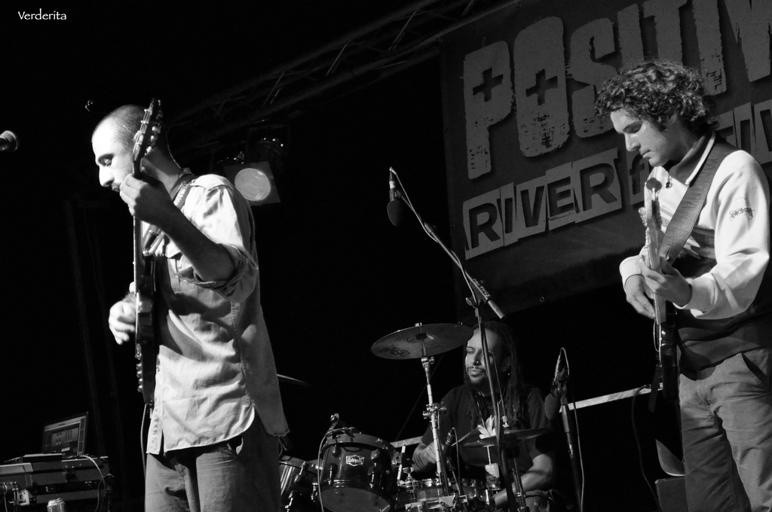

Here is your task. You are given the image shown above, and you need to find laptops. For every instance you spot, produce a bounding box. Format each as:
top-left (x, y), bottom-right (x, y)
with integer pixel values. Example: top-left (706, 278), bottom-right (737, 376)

top-left (40, 411), bottom-right (89, 459)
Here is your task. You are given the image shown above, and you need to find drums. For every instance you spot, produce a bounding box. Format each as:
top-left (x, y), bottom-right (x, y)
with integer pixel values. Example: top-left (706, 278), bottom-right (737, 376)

top-left (311, 430), bottom-right (393, 512)
top-left (397, 479), bottom-right (460, 512)
top-left (276, 452), bottom-right (316, 511)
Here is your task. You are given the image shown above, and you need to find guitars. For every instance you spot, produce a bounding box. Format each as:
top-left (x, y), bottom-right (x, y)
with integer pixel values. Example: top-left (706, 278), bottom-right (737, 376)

top-left (638, 176), bottom-right (679, 425)
top-left (130, 96), bottom-right (164, 407)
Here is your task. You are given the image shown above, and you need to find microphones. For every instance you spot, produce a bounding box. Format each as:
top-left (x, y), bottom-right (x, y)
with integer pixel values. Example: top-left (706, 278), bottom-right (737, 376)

top-left (544, 367), bottom-right (568, 421)
top-left (387, 169), bottom-right (409, 228)
top-left (443, 431), bottom-right (454, 458)
top-left (0, 129), bottom-right (22, 152)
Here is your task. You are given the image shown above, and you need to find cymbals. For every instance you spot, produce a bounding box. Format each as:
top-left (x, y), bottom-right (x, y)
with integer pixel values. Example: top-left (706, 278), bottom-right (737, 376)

top-left (461, 426), bottom-right (546, 449)
top-left (370, 322), bottom-right (475, 361)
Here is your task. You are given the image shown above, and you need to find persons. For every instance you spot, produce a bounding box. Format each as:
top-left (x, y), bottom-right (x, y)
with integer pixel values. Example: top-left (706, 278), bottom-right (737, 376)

top-left (408, 318), bottom-right (557, 512)
top-left (90, 101), bottom-right (295, 511)
top-left (590, 56), bottom-right (770, 512)
